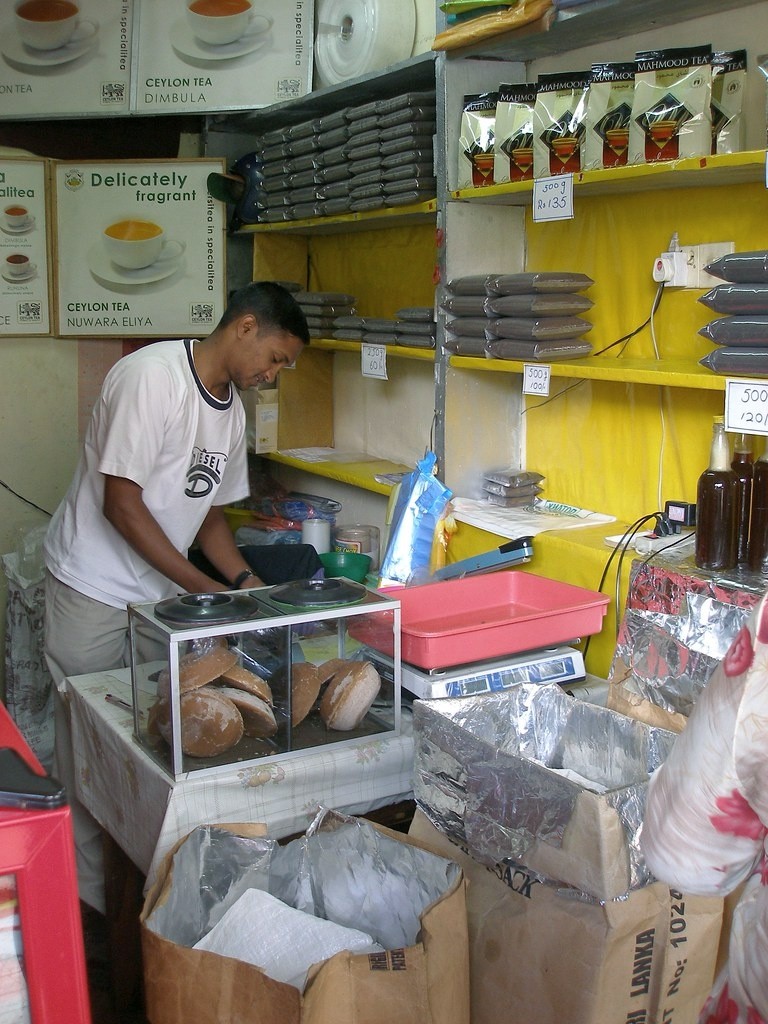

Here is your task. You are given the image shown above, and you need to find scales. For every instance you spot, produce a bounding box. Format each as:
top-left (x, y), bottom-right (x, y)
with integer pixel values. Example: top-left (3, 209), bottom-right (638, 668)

top-left (357, 640), bottom-right (588, 715)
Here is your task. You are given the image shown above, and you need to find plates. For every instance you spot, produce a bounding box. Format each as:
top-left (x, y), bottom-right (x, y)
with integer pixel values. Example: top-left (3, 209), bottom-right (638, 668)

top-left (2, 268), bottom-right (37, 281)
top-left (0, 11), bottom-right (98, 66)
top-left (168, 14), bottom-right (271, 60)
top-left (88, 240), bottom-right (182, 286)
top-left (0, 214), bottom-right (33, 233)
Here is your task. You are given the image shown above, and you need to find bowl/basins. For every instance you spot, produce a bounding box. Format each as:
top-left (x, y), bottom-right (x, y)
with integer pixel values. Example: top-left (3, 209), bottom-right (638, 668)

top-left (317, 552), bottom-right (371, 583)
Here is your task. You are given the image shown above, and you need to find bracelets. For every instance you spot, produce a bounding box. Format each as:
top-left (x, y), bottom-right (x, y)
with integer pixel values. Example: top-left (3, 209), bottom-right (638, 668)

top-left (233, 568), bottom-right (257, 587)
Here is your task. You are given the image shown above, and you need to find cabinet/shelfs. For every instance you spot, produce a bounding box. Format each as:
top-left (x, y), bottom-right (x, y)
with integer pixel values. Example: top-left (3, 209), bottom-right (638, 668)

top-left (236, 0), bottom-right (767, 601)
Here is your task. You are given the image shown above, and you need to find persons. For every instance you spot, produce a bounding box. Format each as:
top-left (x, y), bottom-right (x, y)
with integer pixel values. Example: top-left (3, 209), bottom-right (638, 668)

top-left (643, 581), bottom-right (768, 1024)
top-left (44, 281), bottom-right (311, 972)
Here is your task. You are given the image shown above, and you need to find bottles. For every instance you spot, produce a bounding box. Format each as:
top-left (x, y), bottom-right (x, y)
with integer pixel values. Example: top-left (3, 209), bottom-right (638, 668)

top-left (695, 415), bottom-right (739, 570)
top-left (335, 523), bottom-right (379, 570)
top-left (729, 430), bottom-right (754, 563)
top-left (746, 450), bottom-right (768, 573)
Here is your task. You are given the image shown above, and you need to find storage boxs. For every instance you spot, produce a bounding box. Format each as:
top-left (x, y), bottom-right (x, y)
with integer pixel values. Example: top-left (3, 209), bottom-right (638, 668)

top-left (348, 570), bottom-right (610, 667)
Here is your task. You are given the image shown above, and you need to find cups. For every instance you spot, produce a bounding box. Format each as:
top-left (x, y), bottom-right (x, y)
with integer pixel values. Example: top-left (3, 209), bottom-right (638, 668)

top-left (14, 0), bottom-right (100, 50)
top-left (103, 219), bottom-right (187, 268)
top-left (185, 0), bottom-right (273, 44)
top-left (7, 254), bottom-right (38, 274)
top-left (2, 204), bottom-right (35, 227)
